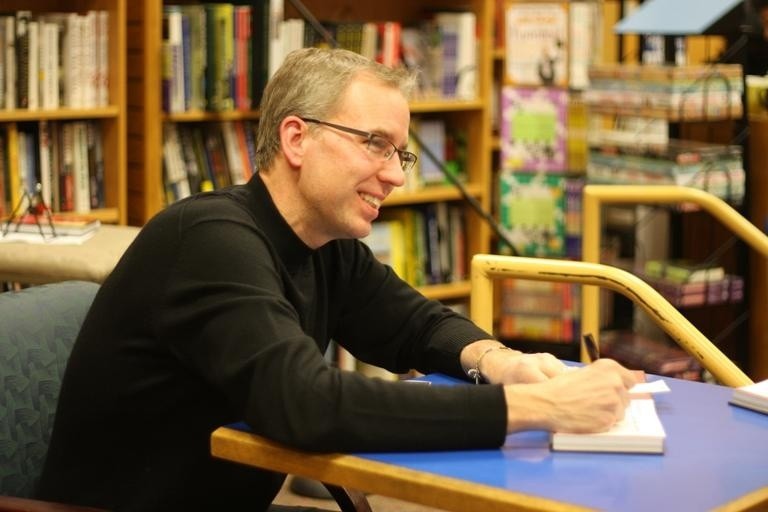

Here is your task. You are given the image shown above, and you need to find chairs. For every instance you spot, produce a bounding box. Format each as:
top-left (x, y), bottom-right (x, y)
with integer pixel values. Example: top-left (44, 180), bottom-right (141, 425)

top-left (0, 280), bottom-right (110, 511)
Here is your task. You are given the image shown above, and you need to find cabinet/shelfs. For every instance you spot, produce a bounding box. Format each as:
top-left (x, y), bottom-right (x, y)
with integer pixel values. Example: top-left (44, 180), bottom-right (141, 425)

top-left (127, 0), bottom-right (492, 321)
top-left (579, 72), bottom-right (749, 311)
top-left (0, 0), bottom-right (127, 228)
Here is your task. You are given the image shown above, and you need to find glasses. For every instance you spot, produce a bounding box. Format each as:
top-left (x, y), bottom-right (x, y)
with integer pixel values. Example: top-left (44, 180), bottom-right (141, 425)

top-left (300, 117), bottom-right (417, 173)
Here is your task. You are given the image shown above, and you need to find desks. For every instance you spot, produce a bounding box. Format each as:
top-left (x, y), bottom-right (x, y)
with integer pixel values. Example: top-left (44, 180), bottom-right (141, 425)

top-left (211, 360), bottom-right (767, 512)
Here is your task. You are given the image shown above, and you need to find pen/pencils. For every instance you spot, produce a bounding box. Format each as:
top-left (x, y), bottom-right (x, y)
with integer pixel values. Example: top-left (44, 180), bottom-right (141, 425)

top-left (584, 335), bottom-right (599, 361)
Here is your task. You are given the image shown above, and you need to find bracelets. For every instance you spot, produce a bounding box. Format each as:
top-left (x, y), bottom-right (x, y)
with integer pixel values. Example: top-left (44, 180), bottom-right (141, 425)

top-left (466, 344), bottom-right (517, 384)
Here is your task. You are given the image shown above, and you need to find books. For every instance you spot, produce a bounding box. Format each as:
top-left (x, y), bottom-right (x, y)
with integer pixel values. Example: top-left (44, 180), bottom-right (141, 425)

top-left (163, 121), bottom-right (258, 206)
top-left (0, 121), bottom-right (105, 224)
top-left (1, 11), bottom-right (109, 109)
top-left (356, 203), bottom-right (471, 285)
top-left (642, 0), bottom-right (687, 66)
top-left (162, 0), bottom-right (480, 114)
top-left (547, 367), bottom-right (666, 456)
top-left (499, 1), bottom-right (605, 338)
top-left (400, 117), bottom-right (445, 189)
top-left (582, 62), bottom-right (745, 385)
top-left (726, 377), bottom-right (768, 416)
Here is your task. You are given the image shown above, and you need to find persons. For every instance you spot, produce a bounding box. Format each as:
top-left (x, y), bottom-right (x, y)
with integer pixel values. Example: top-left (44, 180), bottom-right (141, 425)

top-left (37, 46), bottom-right (640, 512)
